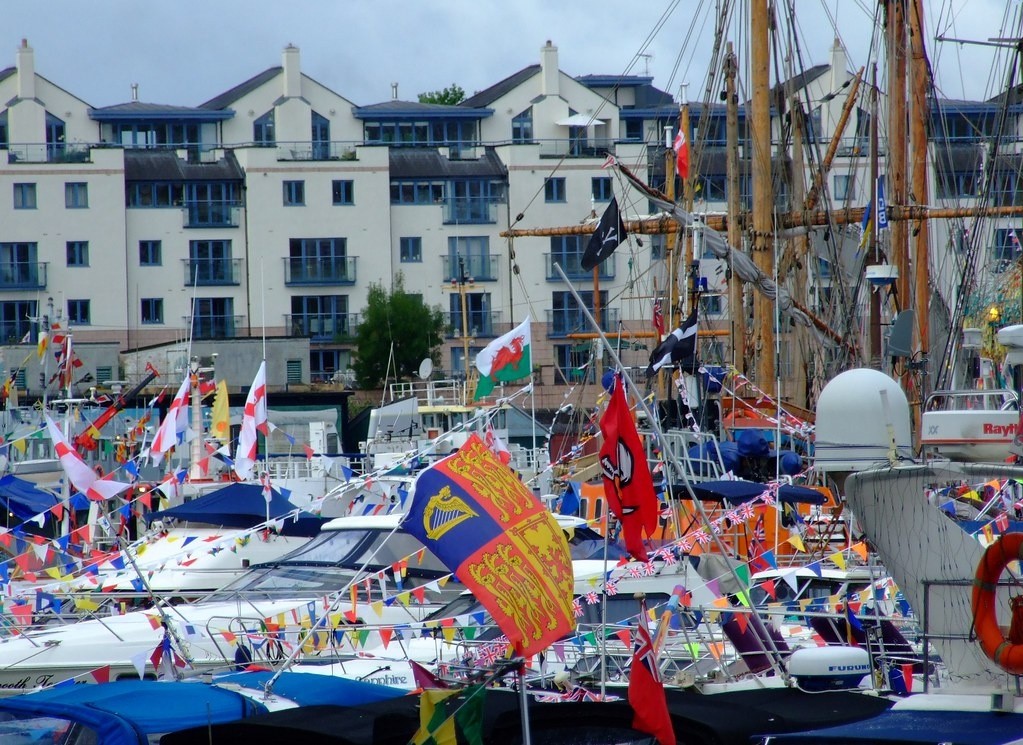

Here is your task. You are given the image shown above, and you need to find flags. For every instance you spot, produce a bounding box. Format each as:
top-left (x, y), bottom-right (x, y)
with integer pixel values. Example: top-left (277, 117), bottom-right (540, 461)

top-left (691, 258), bottom-right (728, 291)
top-left (407, 659), bottom-right (485, 745)
top-left (854, 174), bottom-right (888, 260)
top-left (672, 122), bottom-right (689, 179)
top-left (628, 600), bottom-right (676, 745)
top-left (645, 308), bottom-right (697, 377)
top-left (599, 375), bottom-right (658, 562)
top-left (43, 410), bottom-right (133, 501)
top-left (580, 197), bottom-right (628, 272)
top-left (398, 431), bottom-right (576, 658)
top-left (473, 315), bottom-right (530, 403)
top-left (149, 376), bottom-right (190, 467)
top-left (234, 360), bottom-right (267, 482)
top-left (650, 288), bottom-right (665, 342)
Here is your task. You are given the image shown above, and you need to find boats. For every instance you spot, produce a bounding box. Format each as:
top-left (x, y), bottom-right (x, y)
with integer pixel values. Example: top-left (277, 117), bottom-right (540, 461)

top-left (2, 0), bottom-right (1023, 745)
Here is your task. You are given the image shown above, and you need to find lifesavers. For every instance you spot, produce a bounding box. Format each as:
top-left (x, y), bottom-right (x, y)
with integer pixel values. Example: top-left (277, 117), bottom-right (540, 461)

top-left (970, 531), bottom-right (1022, 677)
